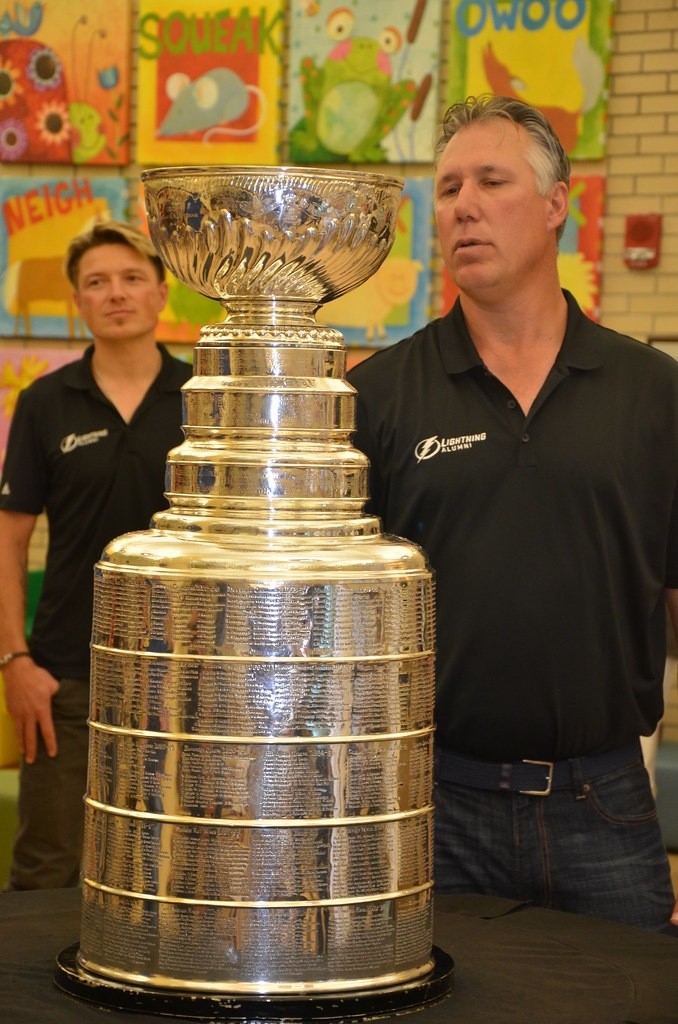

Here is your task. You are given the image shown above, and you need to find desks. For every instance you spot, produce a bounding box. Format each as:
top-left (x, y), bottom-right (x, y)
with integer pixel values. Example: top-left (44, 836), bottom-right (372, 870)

top-left (0, 887), bottom-right (678, 1024)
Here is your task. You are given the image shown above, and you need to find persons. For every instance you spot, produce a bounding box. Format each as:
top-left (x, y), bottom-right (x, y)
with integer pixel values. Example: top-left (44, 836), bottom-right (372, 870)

top-left (346, 95), bottom-right (678, 928)
top-left (0, 222), bottom-right (192, 892)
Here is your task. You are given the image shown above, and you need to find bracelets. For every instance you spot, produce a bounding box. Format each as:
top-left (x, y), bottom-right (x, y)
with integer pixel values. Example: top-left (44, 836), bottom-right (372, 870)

top-left (0, 652), bottom-right (30, 670)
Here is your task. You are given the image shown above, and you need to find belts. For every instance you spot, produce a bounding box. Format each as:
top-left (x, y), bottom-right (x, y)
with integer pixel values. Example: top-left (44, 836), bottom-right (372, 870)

top-left (435, 739), bottom-right (643, 795)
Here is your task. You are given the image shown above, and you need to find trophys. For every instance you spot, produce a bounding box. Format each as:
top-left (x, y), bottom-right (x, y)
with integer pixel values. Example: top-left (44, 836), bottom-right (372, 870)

top-left (59, 166), bottom-right (455, 1024)
